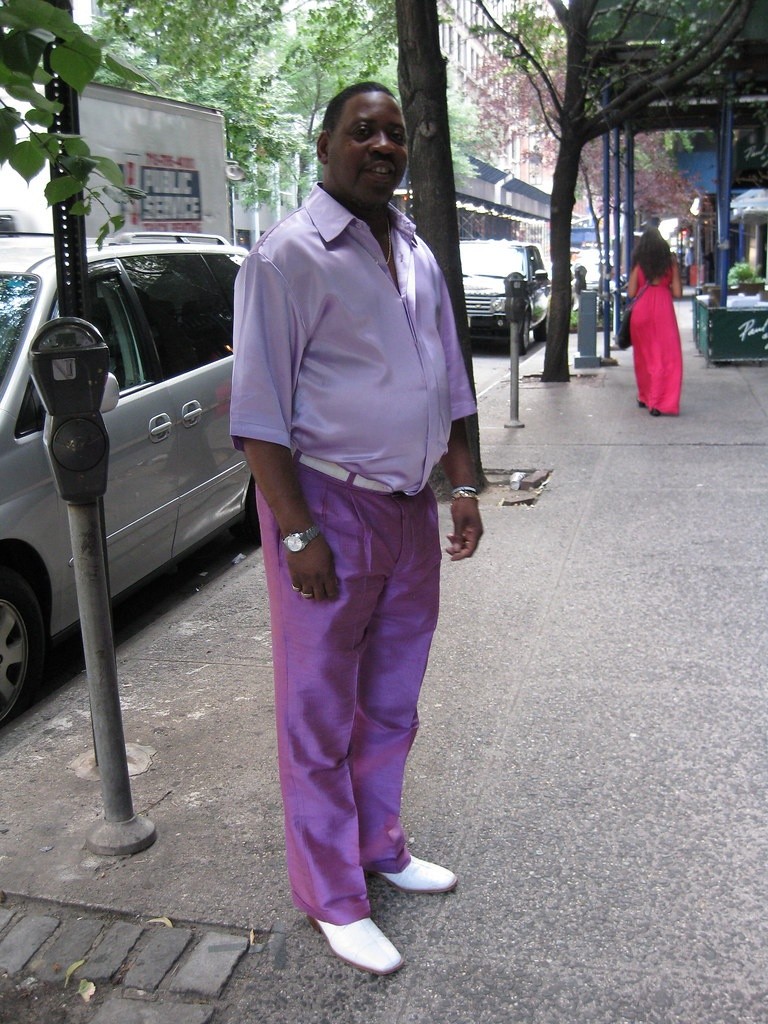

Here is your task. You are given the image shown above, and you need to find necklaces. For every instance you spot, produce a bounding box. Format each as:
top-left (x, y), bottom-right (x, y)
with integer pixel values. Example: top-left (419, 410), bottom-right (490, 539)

top-left (386, 217), bottom-right (391, 263)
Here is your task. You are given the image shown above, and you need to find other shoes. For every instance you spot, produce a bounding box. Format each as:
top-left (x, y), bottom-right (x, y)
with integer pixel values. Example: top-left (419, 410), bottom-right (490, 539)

top-left (379, 854), bottom-right (456, 894)
top-left (308, 914), bottom-right (405, 974)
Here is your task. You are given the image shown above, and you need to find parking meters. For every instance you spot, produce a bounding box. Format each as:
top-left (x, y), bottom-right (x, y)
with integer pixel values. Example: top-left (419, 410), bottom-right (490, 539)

top-left (25, 311), bottom-right (156, 854)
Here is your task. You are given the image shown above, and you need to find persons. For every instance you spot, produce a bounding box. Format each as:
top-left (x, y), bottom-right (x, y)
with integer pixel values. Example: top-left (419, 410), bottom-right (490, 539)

top-left (685, 247), bottom-right (695, 285)
top-left (230, 82), bottom-right (483, 975)
top-left (627, 228), bottom-right (682, 416)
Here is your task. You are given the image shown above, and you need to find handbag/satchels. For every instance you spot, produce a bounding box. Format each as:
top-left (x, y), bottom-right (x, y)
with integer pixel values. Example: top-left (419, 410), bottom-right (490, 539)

top-left (615, 308), bottom-right (633, 347)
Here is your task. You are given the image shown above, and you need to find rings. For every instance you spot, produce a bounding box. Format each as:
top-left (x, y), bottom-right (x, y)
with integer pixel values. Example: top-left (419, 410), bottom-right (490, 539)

top-left (291, 583), bottom-right (302, 591)
top-left (465, 541), bottom-right (469, 543)
top-left (301, 592), bottom-right (313, 598)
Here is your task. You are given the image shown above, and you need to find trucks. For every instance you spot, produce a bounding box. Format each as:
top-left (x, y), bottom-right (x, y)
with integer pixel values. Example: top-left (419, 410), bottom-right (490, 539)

top-left (0, 76), bottom-right (237, 253)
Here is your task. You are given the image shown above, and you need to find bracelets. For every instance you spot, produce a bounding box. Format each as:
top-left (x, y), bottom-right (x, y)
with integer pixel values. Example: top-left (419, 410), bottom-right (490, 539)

top-left (451, 486), bottom-right (478, 499)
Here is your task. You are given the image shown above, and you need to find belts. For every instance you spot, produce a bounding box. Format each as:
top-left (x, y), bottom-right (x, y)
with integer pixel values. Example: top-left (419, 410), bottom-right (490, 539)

top-left (292, 452), bottom-right (401, 497)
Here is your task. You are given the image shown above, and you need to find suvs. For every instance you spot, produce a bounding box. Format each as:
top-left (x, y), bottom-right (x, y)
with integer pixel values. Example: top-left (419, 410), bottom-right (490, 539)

top-left (1, 228), bottom-right (257, 738)
top-left (456, 238), bottom-right (555, 357)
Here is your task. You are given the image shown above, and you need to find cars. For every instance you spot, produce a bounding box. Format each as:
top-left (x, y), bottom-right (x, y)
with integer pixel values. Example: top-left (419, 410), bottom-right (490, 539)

top-left (568, 246), bottom-right (614, 292)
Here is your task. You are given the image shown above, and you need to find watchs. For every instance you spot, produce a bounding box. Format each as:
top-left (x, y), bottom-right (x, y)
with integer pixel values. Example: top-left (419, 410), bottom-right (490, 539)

top-left (281, 525), bottom-right (319, 551)
